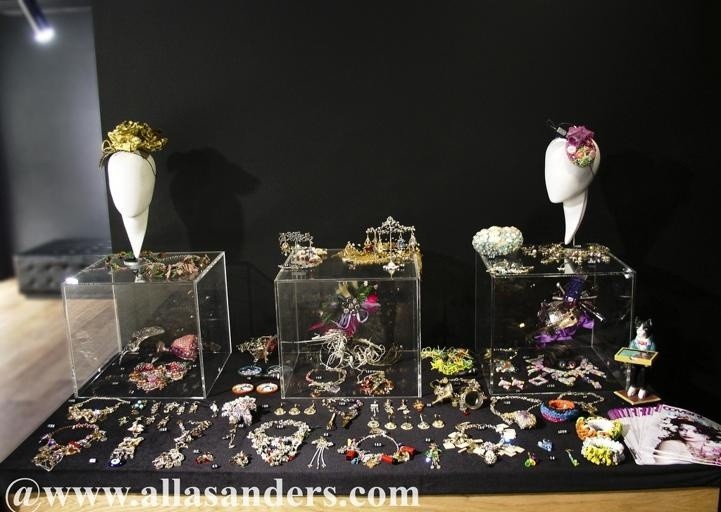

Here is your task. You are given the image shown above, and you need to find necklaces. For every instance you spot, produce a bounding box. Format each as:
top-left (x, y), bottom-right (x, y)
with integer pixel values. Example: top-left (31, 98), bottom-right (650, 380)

top-left (68, 397), bottom-right (131, 424)
top-left (555, 391), bottom-right (606, 418)
top-left (441, 421), bottom-right (527, 466)
top-left (489, 395), bottom-right (542, 430)
top-left (32, 422), bottom-right (108, 473)
top-left (337, 434), bottom-right (417, 471)
top-left (245, 419), bottom-right (311, 467)
top-left (306, 367), bottom-right (347, 398)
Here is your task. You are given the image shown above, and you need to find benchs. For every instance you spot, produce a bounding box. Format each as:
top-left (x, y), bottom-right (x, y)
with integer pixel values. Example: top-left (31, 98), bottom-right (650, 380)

top-left (12, 236), bottom-right (117, 301)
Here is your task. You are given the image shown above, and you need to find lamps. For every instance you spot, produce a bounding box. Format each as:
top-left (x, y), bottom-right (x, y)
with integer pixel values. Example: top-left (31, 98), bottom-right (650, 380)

top-left (18, 0), bottom-right (55, 44)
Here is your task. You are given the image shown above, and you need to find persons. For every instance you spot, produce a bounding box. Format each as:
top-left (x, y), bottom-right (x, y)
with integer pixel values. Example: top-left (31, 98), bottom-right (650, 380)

top-left (656, 417), bottom-right (719, 461)
top-left (623, 316), bottom-right (655, 400)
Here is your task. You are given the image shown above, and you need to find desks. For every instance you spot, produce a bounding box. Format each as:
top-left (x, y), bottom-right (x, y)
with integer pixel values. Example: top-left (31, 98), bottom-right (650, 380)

top-left (0, 349), bottom-right (721, 512)
